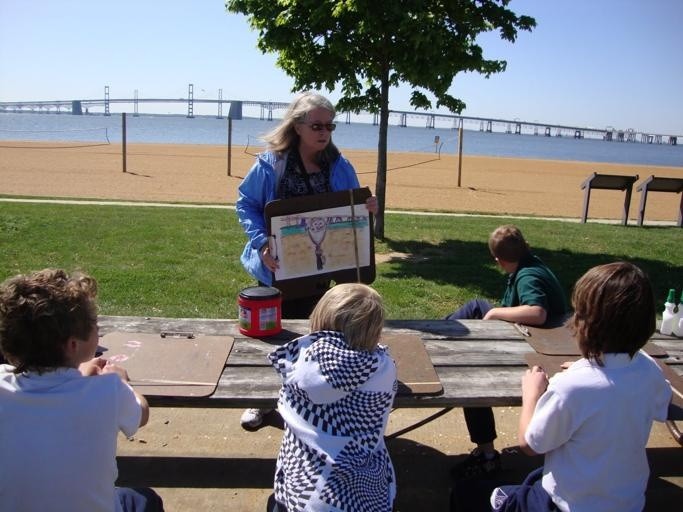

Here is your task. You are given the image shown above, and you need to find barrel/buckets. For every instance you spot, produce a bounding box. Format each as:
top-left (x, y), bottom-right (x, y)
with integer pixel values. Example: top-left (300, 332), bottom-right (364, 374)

top-left (237, 286), bottom-right (282, 337)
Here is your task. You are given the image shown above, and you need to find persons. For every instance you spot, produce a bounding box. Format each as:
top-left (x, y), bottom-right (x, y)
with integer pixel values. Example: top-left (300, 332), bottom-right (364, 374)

top-left (450, 262), bottom-right (676, 512)
top-left (443, 225), bottom-right (567, 472)
top-left (267, 282), bottom-right (399, 511)
top-left (0, 267), bottom-right (166, 512)
top-left (234, 91), bottom-right (379, 430)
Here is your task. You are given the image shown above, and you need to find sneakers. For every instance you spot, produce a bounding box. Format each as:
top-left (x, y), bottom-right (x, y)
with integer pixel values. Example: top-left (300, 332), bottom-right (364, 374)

top-left (451, 448), bottom-right (503, 484)
top-left (239, 406), bottom-right (274, 432)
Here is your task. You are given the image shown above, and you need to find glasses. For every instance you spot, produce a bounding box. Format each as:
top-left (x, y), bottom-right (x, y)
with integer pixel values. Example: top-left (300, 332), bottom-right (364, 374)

top-left (309, 123), bottom-right (336, 132)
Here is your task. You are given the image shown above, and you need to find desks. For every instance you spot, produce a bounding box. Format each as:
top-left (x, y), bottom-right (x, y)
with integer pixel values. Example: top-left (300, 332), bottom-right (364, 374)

top-left (85, 308), bottom-right (683, 468)
